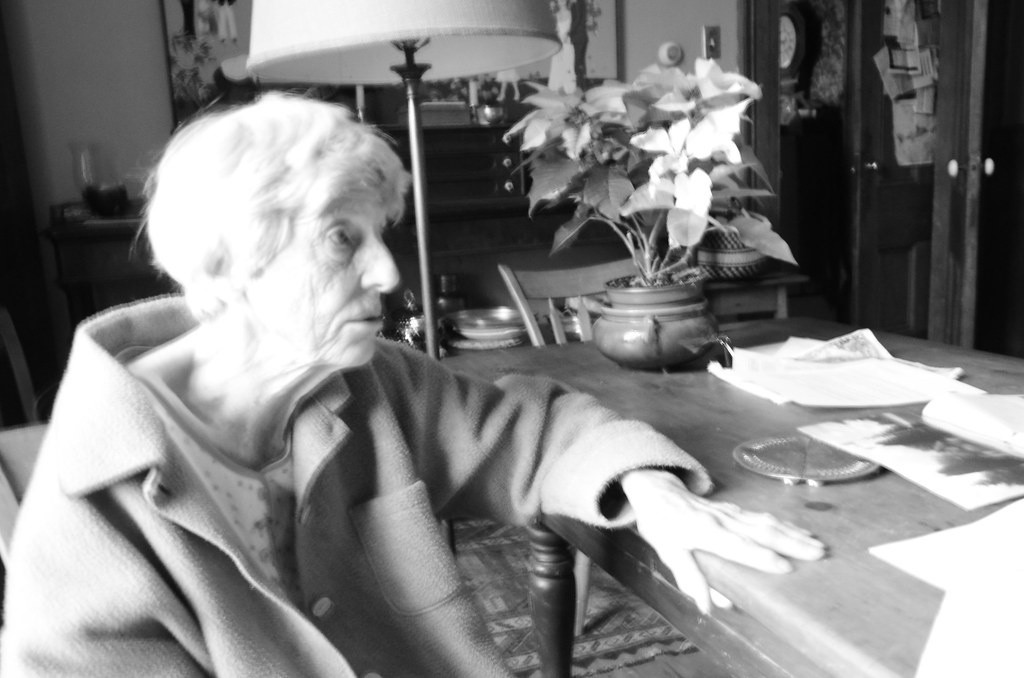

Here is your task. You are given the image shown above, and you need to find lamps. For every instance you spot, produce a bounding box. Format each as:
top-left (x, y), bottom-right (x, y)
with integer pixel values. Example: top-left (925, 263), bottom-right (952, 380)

top-left (246, 1), bottom-right (564, 355)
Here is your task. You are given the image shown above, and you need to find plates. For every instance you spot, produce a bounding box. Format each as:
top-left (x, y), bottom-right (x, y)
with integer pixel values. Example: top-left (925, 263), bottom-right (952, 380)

top-left (734, 437), bottom-right (880, 480)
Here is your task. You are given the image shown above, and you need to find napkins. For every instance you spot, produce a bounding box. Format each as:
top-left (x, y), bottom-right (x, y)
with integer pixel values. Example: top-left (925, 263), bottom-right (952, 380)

top-left (706, 328), bottom-right (1024, 589)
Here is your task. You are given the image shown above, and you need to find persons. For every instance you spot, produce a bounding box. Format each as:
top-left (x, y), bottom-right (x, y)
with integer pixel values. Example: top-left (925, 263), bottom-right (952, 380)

top-left (0, 89), bottom-right (830, 678)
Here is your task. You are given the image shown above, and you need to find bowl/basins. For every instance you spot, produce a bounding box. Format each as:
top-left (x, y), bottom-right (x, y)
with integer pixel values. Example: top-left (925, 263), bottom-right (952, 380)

top-left (444, 307), bottom-right (537, 340)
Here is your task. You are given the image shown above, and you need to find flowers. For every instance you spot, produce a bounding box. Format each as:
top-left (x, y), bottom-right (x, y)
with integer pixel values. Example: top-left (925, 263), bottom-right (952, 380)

top-left (512, 57), bottom-right (799, 286)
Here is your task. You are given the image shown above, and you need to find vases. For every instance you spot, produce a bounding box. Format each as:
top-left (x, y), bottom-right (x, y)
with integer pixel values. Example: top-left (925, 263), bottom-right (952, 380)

top-left (593, 269), bottom-right (721, 372)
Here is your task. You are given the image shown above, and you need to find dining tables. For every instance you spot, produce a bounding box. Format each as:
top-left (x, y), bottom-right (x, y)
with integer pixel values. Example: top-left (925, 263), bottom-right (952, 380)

top-left (427, 315), bottom-right (1024, 677)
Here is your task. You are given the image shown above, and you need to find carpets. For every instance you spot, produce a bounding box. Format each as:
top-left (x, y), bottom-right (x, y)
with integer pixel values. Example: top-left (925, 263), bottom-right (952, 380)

top-left (437, 518), bottom-right (698, 677)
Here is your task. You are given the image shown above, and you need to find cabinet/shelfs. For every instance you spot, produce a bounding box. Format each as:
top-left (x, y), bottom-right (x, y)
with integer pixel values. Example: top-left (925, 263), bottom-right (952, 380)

top-left (52, 199), bottom-right (181, 328)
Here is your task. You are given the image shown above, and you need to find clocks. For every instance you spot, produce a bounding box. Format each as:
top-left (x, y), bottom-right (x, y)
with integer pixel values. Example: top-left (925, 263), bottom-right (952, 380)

top-left (778, 0), bottom-right (816, 127)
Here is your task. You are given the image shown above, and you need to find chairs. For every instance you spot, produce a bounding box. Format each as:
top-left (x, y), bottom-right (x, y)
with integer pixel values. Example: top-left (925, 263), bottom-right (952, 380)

top-left (498, 254), bottom-right (642, 347)
